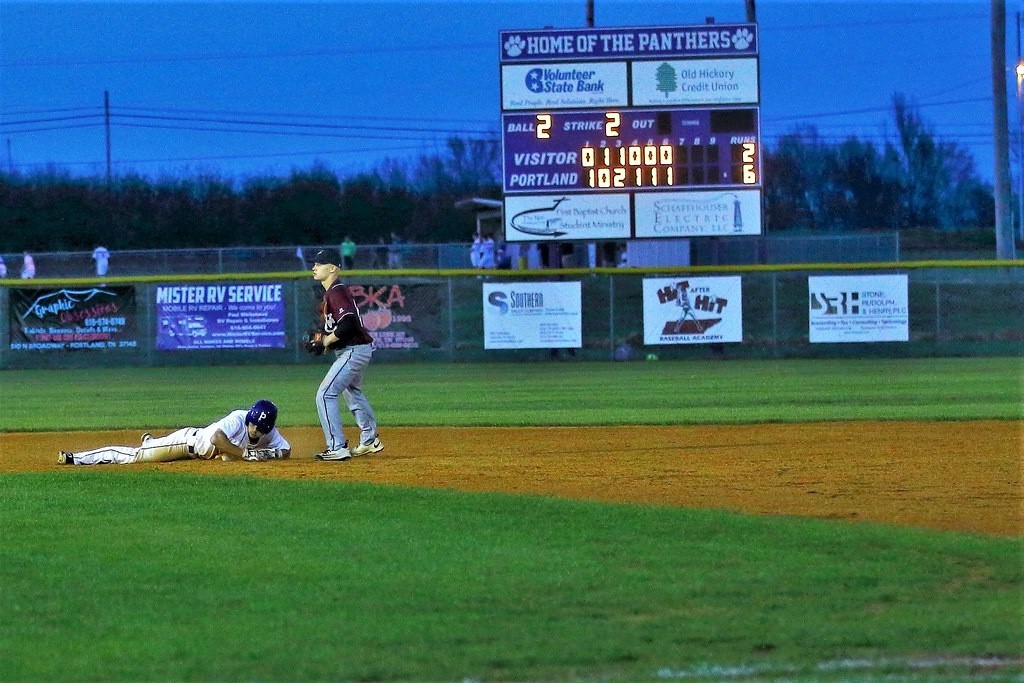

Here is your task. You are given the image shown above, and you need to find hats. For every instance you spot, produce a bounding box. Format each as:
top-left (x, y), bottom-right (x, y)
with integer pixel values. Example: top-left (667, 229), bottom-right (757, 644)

top-left (306, 248), bottom-right (342, 268)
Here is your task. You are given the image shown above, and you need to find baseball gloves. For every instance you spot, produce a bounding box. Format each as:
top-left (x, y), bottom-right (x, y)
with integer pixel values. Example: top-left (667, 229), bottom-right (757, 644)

top-left (303, 327), bottom-right (326, 356)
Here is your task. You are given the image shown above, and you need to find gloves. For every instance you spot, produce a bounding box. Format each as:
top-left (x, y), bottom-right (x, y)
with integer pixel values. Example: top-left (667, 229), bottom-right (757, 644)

top-left (242, 447), bottom-right (268, 462)
top-left (259, 447), bottom-right (283, 459)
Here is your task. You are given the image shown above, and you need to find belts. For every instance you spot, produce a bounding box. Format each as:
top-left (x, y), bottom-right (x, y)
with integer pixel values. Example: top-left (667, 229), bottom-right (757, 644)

top-left (189, 431), bottom-right (196, 454)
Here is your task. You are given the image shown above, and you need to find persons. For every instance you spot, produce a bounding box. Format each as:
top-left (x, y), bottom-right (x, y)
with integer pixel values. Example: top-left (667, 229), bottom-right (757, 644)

top-left (336, 231), bottom-right (411, 270)
top-left (304, 248), bottom-right (385, 462)
top-left (469, 232), bottom-right (632, 270)
top-left (0, 258), bottom-right (7, 278)
top-left (18, 250), bottom-right (37, 279)
top-left (92, 241), bottom-right (110, 275)
top-left (56, 399), bottom-right (292, 466)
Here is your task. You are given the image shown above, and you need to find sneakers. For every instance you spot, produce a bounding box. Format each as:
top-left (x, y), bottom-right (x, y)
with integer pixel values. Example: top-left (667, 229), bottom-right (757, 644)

top-left (141, 432), bottom-right (153, 444)
top-left (58, 450), bottom-right (74, 465)
top-left (350, 434), bottom-right (384, 457)
top-left (313, 440), bottom-right (351, 461)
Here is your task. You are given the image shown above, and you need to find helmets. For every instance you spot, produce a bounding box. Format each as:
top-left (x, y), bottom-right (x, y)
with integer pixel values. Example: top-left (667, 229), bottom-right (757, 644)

top-left (245, 400), bottom-right (277, 434)
